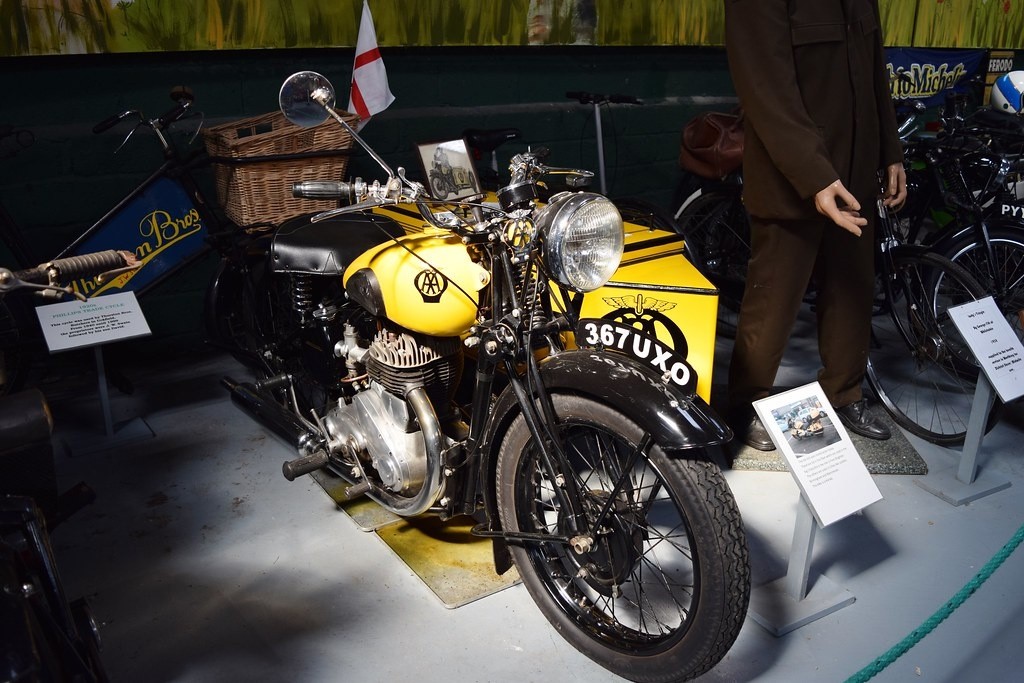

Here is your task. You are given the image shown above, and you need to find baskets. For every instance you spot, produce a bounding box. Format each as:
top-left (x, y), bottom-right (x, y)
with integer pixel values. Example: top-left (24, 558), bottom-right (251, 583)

top-left (202, 105), bottom-right (361, 233)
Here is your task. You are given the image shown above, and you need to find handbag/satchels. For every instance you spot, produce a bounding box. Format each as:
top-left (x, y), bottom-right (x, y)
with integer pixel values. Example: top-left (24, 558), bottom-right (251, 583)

top-left (678, 112), bottom-right (743, 177)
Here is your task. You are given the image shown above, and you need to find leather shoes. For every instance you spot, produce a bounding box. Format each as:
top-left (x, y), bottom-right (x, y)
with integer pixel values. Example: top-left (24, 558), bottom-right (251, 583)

top-left (727, 400), bottom-right (775, 451)
top-left (834, 399), bottom-right (891, 440)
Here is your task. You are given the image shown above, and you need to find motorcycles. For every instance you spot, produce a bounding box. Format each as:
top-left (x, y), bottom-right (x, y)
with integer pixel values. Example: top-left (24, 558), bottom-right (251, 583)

top-left (0, 248), bottom-right (144, 683)
top-left (218, 72), bottom-right (752, 683)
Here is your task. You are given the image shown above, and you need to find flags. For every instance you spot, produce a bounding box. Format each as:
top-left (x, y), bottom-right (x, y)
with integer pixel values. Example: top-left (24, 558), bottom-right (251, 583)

top-left (347, 0), bottom-right (396, 134)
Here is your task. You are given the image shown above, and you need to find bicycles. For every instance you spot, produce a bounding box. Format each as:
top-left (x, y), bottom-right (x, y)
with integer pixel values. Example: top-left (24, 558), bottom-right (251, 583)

top-left (461, 76), bottom-right (1023, 446)
top-left (1, 85), bottom-right (292, 365)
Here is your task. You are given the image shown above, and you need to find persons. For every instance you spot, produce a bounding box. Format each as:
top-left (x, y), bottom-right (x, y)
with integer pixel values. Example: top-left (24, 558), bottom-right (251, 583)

top-left (720, 0), bottom-right (908, 451)
top-left (435, 147), bottom-right (449, 166)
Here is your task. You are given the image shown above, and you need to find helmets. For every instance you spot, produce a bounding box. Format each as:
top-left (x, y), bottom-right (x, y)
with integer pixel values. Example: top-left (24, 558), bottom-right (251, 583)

top-left (990, 70), bottom-right (1024, 114)
